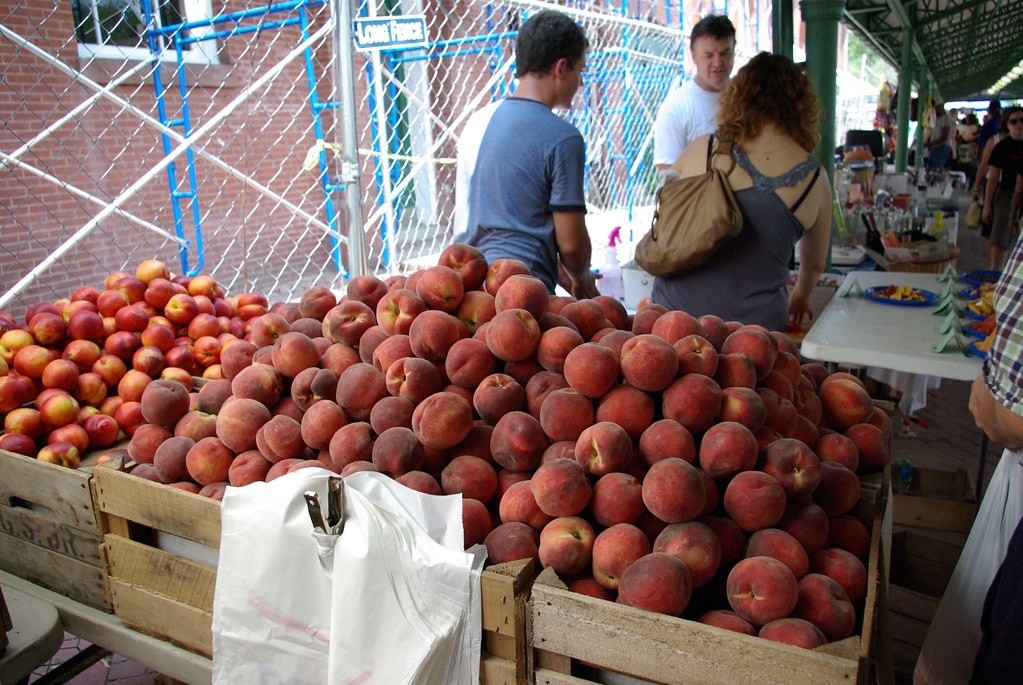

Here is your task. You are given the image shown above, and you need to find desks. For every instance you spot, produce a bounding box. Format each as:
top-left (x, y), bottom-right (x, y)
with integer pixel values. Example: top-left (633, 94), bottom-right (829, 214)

top-left (799, 165), bottom-right (990, 511)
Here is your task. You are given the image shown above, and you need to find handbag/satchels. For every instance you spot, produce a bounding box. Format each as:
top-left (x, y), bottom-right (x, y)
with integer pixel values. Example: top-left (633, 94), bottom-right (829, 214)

top-left (930, 118), bottom-right (950, 145)
top-left (635, 140), bottom-right (743, 277)
top-left (964, 199), bottom-right (982, 228)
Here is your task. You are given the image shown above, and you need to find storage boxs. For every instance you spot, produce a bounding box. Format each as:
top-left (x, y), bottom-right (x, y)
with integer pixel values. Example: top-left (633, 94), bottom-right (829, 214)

top-left (0, 398), bottom-right (898, 684)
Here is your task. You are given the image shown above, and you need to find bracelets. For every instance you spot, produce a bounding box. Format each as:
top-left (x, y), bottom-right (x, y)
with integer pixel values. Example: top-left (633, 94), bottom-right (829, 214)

top-left (972, 187), bottom-right (980, 191)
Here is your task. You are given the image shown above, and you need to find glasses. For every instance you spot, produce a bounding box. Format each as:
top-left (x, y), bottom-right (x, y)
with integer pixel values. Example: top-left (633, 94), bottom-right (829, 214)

top-left (1008, 116), bottom-right (1023, 125)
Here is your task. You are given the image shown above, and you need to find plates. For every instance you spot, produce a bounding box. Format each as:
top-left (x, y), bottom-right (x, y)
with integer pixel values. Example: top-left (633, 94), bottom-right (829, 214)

top-left (866, 284), bottom-right (941, 307)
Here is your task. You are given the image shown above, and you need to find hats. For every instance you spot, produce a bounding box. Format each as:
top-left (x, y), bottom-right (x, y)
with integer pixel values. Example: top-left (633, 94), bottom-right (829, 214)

top-left (985, 100), bottom-right (1001, 114)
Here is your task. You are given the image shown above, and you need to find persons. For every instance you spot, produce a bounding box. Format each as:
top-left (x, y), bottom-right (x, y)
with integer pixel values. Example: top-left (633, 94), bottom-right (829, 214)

top-left (651, 51), bottom-right (833, 333)
top-left (924, 100), bottom-right (1023, 271)
top-left (449, 10), bottom-right (600, 301)
top-left (653, 15), bottom-right (736, 179)
top-left (913, 227), bottom-right (1023, 685)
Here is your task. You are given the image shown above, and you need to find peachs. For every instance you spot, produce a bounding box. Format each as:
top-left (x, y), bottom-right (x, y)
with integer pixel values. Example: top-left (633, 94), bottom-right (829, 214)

top-left (888, 282), bottom-right (921, 301)
top-left (0, 260), bottom-right (290, 466)
top-left (122, 245), bottom-right (891, 650)
